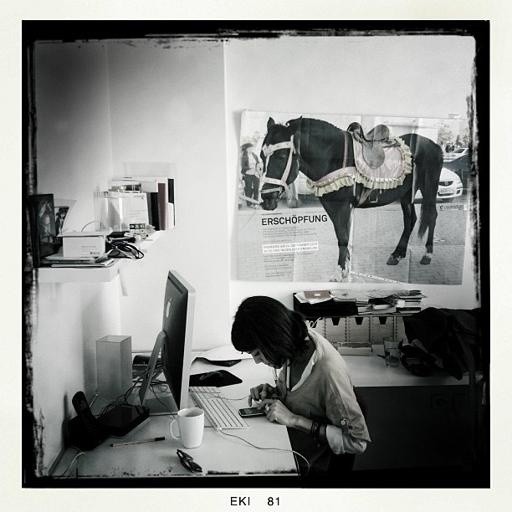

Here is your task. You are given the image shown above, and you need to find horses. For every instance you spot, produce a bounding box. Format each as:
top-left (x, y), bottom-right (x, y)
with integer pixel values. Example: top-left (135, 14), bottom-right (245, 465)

top-left (259, 116), bottom-right (444, 282)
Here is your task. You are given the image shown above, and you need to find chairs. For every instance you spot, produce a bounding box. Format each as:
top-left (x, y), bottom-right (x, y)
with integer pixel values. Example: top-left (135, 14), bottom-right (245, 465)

top-left (300, 387), bottom-right (366, 479)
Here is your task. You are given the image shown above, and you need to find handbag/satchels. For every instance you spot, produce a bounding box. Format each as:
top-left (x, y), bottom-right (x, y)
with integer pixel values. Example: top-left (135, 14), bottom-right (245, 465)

top-left (404, 309), bottom-right (473, 381)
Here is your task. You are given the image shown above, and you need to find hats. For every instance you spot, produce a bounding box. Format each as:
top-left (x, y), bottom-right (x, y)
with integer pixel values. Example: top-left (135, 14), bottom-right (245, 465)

top-left (399, 340), bottom-right (445, 378)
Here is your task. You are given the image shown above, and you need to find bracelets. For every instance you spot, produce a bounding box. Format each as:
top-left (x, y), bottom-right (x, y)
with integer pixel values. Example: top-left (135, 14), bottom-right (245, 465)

top-left (310, 421), bottom-right (322, 439)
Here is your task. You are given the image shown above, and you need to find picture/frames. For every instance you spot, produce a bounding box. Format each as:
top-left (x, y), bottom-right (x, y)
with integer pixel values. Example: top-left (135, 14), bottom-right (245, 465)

top-left (30, 192), bottom-right (56, 258)
top-left (51, 205), bottom-right (68, 247)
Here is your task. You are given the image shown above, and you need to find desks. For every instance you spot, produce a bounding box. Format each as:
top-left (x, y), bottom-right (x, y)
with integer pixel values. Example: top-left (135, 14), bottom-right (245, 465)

top-left (61, 341), bottom-right (484, 479)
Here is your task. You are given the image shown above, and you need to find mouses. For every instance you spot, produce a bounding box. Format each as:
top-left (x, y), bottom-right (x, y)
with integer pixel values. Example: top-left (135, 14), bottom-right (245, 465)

top-left (199, 372), bottom-right (224, 381)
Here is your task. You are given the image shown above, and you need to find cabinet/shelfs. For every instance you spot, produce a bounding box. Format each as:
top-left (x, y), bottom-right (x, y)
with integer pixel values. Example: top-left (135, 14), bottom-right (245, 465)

top-left (33, 227), bottom-right (169, 284)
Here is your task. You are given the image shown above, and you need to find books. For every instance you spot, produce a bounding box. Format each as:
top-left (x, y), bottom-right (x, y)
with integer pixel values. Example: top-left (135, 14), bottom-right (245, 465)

top-left (101, 176), bottom-right (176, 233)
top-left (294, 290), bottom-right (428, 316)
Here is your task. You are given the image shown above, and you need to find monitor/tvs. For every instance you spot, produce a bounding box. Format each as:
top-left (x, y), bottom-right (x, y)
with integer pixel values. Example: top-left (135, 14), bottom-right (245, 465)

top-left (130, 267), bottom-right (195, 417)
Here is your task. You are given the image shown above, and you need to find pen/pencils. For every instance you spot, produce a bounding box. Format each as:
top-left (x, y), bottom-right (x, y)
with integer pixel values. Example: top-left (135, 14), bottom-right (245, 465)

top-left (111, 437), bottom-right (165, 447)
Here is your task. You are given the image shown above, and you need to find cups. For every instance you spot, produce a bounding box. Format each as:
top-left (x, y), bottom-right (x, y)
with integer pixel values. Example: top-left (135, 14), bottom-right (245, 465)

top-left (169, 407), bottom-right (205, 450)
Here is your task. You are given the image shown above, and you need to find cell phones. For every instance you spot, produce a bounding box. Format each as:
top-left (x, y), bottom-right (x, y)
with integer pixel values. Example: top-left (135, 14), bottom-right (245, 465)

top-left (238, 408), bottom-right (266, 418)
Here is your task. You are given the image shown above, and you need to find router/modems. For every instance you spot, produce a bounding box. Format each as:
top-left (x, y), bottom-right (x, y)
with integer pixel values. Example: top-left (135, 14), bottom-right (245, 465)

top-left (96, 404), bottom-right (149, 436)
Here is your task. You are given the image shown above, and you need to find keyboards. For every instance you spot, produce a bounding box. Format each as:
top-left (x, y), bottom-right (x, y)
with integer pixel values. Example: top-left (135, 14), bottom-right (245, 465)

top-left (190, 386), bottom-right (249, 430)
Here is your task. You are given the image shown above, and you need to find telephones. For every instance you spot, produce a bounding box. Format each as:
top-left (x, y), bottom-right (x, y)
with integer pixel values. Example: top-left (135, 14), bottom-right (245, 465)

top-left (66, 391), bottom-right (109, 452)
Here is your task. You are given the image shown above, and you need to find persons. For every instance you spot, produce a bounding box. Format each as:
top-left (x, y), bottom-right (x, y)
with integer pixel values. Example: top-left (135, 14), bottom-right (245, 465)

top-left (230, 295), bottom-right (374, 456)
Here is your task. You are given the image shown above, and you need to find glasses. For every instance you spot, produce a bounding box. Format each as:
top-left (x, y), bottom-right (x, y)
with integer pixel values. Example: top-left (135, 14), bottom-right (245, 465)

top-left (175, 448), bottom-right (203, 473)
